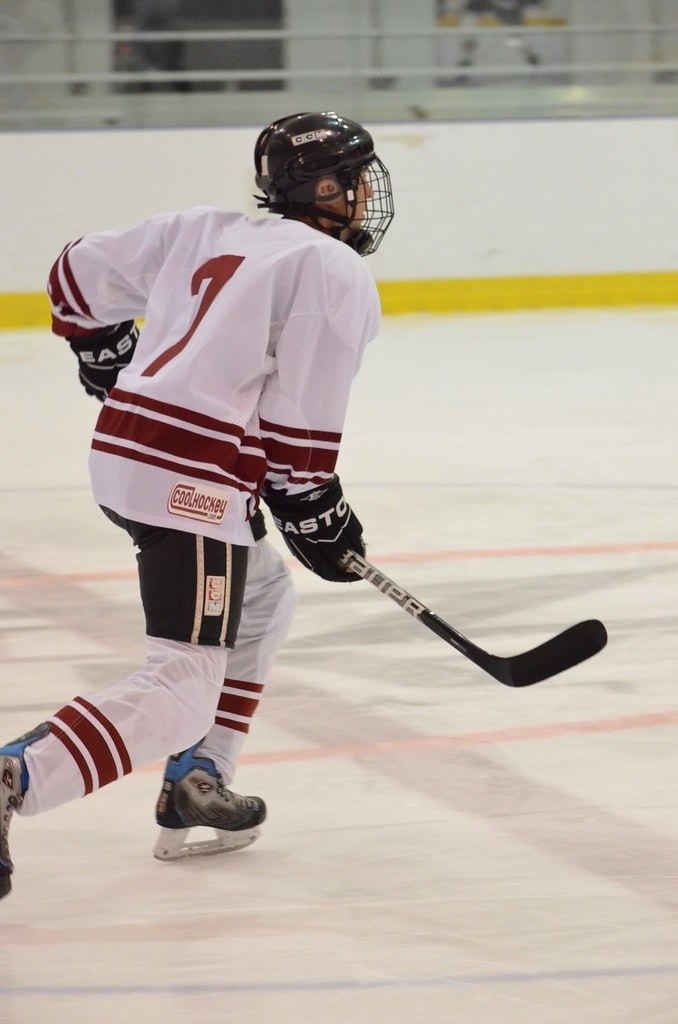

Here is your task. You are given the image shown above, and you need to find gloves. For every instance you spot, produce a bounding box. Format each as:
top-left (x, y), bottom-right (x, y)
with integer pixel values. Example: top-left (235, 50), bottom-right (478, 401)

top-left (65, 319), bottom-right (140, 402)
top-left (258, 474), bottom-right (366, 582)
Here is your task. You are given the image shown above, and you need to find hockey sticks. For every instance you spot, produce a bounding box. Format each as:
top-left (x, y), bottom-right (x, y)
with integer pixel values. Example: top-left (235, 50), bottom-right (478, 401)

top-left (256, 488), bottom-right (609, 689)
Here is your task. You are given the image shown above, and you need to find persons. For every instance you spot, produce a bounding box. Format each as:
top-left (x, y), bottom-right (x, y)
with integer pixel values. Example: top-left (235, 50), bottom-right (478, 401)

top-left (0, 110), bottom-right (398, 899)
top-left (431, 0), bottom-right (580, 91)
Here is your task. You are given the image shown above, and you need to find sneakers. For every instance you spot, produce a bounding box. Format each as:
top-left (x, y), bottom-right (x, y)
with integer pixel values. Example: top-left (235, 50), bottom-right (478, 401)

top-left (0, 721), bottom-right (52, 899)
top-left (151, 737), bottom-right (266, 861)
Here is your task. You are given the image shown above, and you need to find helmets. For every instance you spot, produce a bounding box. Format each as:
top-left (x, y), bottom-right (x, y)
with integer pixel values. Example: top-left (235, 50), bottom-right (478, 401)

top-left (255, 111), bottom-right (394, 258)
